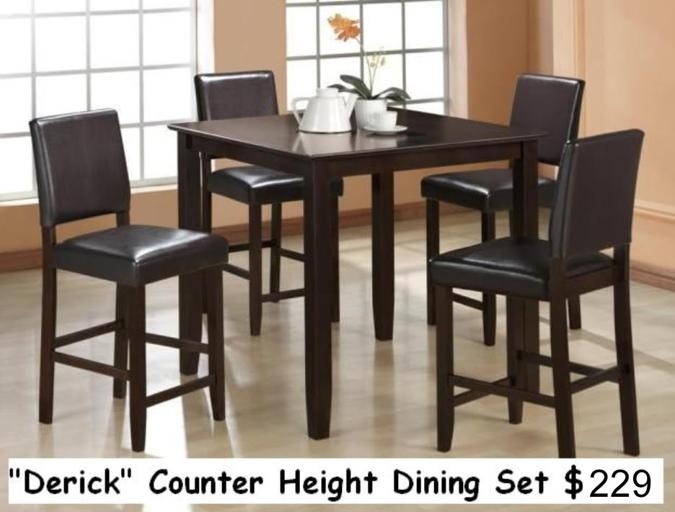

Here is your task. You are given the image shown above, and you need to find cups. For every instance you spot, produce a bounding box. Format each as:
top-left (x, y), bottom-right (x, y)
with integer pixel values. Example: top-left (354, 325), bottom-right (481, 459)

top-left (368, 110), bottom-right (399, 130)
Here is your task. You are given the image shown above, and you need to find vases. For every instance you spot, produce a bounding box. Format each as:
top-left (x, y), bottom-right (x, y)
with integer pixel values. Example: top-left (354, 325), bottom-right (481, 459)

top-left (352, 98), bottom-right (386, 130)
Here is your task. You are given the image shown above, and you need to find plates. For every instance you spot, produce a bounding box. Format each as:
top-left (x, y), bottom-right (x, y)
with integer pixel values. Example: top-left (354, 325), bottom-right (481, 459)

top-left (362, 125), bottom-right (409, 134)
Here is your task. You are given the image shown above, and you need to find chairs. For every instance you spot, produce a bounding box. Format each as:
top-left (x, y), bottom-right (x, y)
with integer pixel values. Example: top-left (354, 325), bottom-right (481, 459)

top-left (193, 70), bottom-right (345, 336)
top-left (27, 107), bottom-right (227, 453)
top-left (420, 70), bottom-right (646, 459)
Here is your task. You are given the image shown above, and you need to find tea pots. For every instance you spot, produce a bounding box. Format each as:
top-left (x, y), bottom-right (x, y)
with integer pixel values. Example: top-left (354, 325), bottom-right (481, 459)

top-left (292, 87), bottom-right (359, 134)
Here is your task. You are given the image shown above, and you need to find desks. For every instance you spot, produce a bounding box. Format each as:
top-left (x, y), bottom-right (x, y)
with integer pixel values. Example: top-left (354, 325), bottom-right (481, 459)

top-left (164, 107), bottom-right (552, 441)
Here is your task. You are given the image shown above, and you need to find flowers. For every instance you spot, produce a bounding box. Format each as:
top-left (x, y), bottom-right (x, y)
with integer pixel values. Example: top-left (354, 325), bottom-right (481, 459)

top-left (325, 13), bottom-right (412, 105)
